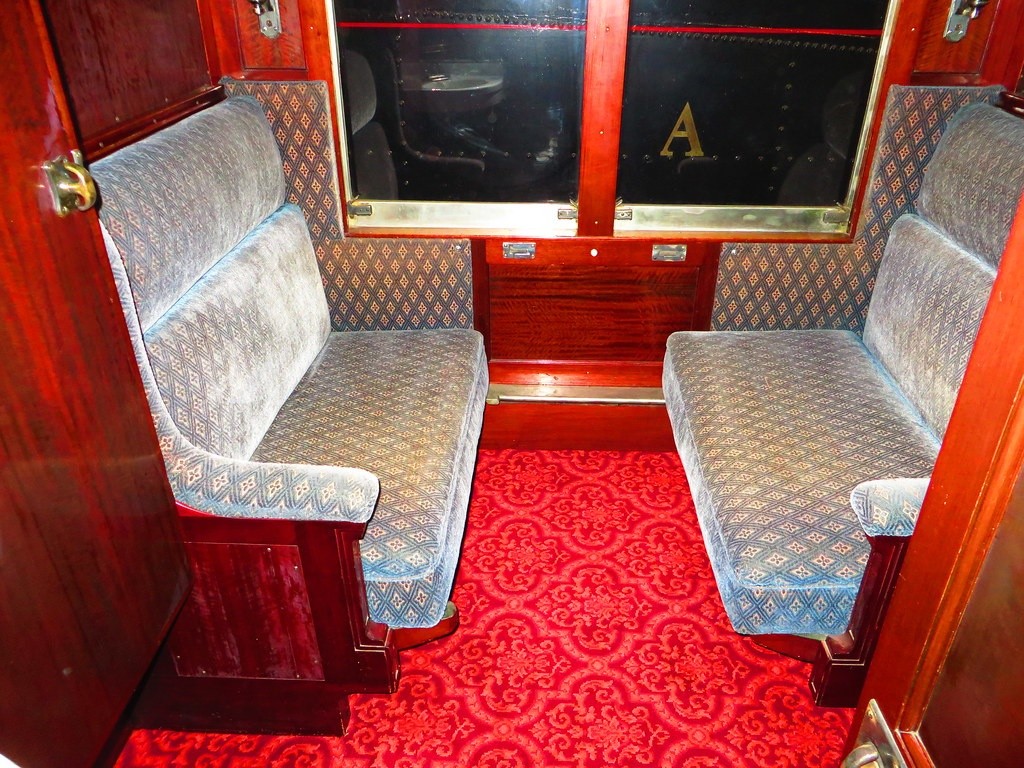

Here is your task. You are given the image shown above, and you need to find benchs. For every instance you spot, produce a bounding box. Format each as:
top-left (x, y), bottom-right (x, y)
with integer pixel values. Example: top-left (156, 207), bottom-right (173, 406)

top-left (661, 99), bottom-right (1024, 707)
top-left (88, 94), bottom-right (489, 740)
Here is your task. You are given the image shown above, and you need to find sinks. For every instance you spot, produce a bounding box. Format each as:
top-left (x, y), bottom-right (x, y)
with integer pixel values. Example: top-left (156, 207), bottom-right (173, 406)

top-left (422, 78), bottom-right (489, 89)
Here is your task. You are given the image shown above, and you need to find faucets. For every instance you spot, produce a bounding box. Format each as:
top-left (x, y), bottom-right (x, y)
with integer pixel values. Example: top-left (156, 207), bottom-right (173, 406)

top-left (424, 50), bottom-right (457, 82)
